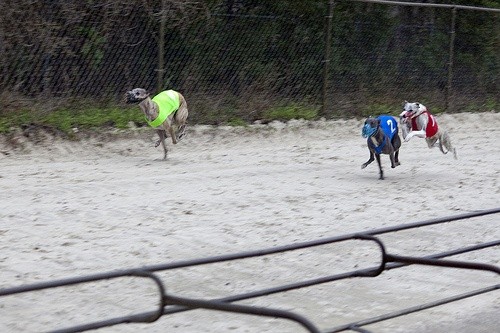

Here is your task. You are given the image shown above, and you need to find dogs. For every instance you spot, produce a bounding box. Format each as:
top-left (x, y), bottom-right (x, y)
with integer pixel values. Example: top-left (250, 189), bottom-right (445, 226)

top-left (123, 87), bottom-right (188, 160)
top-left (360, 115), bottom-right (401, 181)
top-left (396, 100), bottom-right (457, 161)
